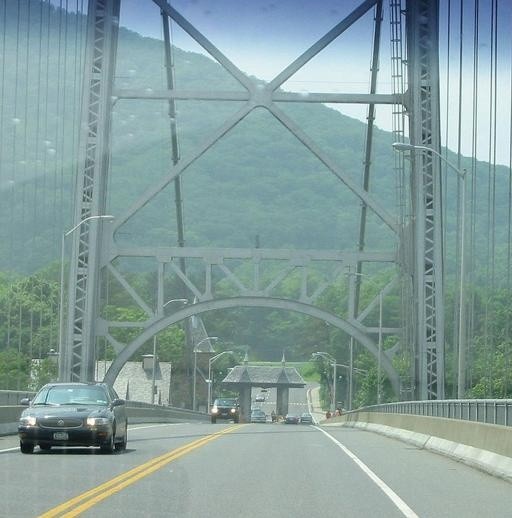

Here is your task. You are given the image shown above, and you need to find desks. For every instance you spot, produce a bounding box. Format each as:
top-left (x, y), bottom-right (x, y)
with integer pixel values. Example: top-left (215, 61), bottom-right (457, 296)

top-left (343, 271), bottom-right (383, 406)
top-left (193, 336), bottom-right (218, 412)
top-left (311, 351), bottom-right (337, 415)
top-left (208, 350), bottom-right (234, 409)
top-left (57, 213), bottom-right (117, 382)
top-left (393, 139), bottom-right (467, 401)
top-left (150, 297), bottom-right (188, 404)
top-left (331, 363), bottom-right (370, 378)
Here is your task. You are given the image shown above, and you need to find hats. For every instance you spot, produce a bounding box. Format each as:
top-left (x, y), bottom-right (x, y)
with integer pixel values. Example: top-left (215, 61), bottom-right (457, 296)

top-left (18, 378), bottom-right (130, 454)
top-left (251, 393), bottom-right (315, 425)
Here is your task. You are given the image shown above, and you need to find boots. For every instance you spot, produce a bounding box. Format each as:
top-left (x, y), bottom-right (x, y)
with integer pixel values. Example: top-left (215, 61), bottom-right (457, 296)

top-left (209, 398), bottom-right (241, 424)
